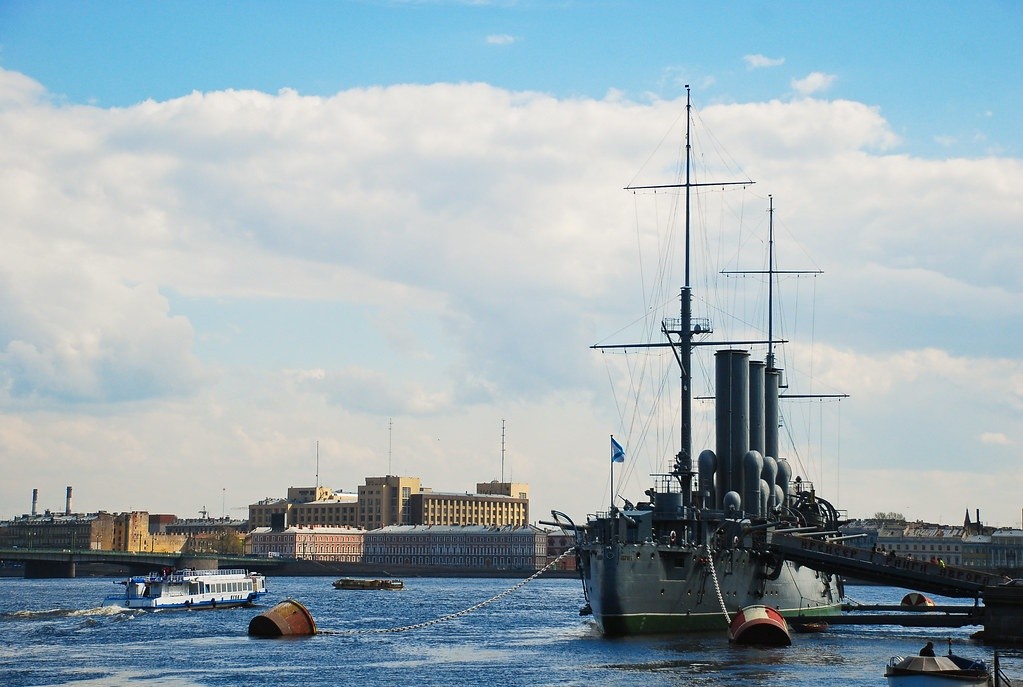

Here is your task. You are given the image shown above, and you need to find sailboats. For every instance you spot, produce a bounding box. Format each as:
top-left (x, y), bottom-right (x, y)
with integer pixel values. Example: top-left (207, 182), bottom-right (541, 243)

top-left (574, 84), bottom-right (851, 637)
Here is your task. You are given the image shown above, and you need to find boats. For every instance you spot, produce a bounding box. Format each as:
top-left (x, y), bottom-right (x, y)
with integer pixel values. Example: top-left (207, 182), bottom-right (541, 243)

top-left (885, 652), bottom-right (994, 687)
top-left (102, 570), bottom-right (267, 610)
top-left (332, 578), bottom-right (404, 590)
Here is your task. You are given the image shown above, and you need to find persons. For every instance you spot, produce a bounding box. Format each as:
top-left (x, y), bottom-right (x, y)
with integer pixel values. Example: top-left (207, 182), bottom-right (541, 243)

top-left (872, 543), bottom-right (897, 558)
top-left (920, 641), bottom-right (936, 657)
top-left (930, 556), bottom-right (945, 569)
top-left (905, 554), bottom-right (912, 561)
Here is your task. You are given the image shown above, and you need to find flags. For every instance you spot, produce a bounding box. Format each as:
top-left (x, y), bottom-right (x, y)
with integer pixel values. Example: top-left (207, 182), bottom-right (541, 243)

top-left (611, 439), bottom-right (625, 462)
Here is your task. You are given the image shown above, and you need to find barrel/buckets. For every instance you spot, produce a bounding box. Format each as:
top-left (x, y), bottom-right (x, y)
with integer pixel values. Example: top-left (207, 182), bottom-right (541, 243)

top-left (654, 492), bottom-right (681, 519)
top-left (901, 593), bottom-right (936, 607)
top-left (729, 605), bottom-right (792, 648)
top-left (248, 599), bottom-right (317, 636)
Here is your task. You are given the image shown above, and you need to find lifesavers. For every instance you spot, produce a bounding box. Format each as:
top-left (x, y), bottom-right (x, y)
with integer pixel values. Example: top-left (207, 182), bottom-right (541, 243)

top-left (734, 536), bottom-right (738, 546)
top-left (670, 530), bottom-right (676, 541)
